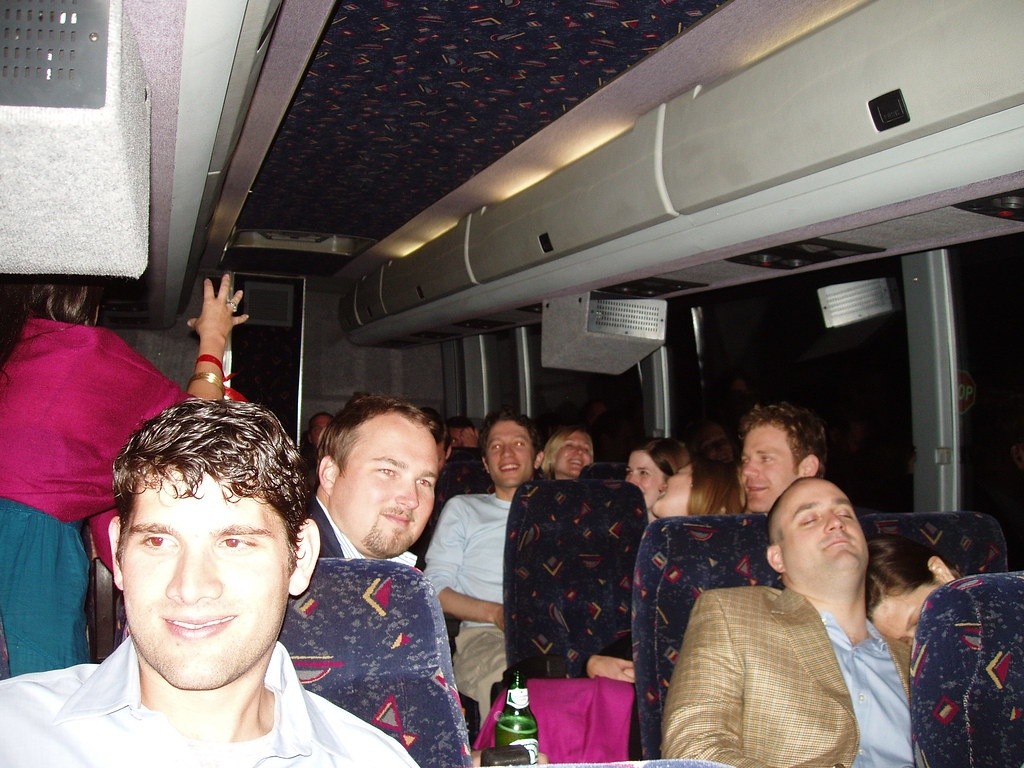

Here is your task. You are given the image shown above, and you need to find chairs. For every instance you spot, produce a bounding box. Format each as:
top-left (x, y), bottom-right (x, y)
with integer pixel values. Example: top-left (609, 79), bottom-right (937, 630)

top-left (91, 423), bottom-right (1024, 768)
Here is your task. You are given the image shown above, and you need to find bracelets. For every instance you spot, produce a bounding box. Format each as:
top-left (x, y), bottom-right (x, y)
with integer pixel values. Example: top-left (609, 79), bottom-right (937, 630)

top-left (186, 371), bottom-right (227, 397)
top-left (194, 354), bottom-right (239, 385)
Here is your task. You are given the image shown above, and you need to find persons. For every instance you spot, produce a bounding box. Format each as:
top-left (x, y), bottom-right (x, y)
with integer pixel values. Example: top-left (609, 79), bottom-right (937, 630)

top-left (0, 258), bottom-right (252, 676)
top-left (732, 394), bottom-right (831, 516)
top-left (617, 436), bottom-right (684, 530)
top-left (850, 515), bottom-right (981, 668)
top-left (296, 412), bottom-right (336, 479)
top-left (266, 390), bottom-right (443, 589)
top-left (415, 407), bottom-right (477, 571)
top-left (471, 454), bottom-right (747, 766)
top-left (0, 399), bottom-right (422, 768)
top-left (442, 415), bottom-right (483, 453)
top-left (652, 469), bottom-right (925, 767)
top-left (415, 411), bottom-right (541, 745)
top-left (530, 428), bottom-right (603, 487)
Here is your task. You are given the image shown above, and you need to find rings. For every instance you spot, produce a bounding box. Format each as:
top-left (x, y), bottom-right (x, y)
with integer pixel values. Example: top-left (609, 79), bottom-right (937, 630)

top-left (225, 299), bottom-right (237, 313)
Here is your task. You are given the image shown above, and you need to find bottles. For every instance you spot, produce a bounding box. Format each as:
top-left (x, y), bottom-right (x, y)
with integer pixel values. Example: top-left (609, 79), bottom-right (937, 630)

top-left (495, 667), bottom-right (539, 766)
